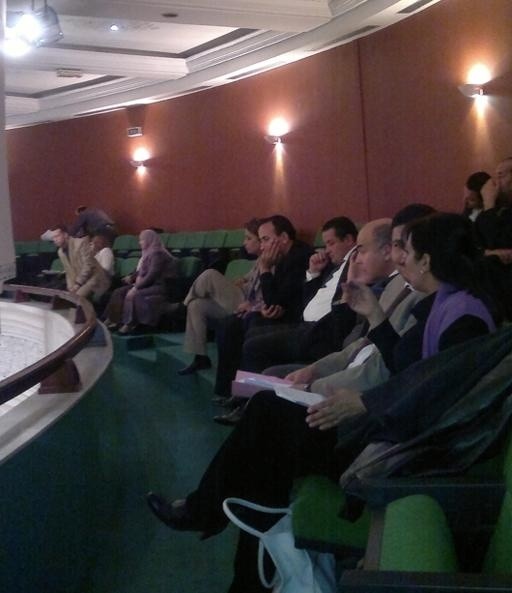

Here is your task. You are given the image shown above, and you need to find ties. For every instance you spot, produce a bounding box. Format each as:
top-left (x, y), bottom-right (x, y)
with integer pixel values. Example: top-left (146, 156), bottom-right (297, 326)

top-left (346, 286), bottom-right (411, 365)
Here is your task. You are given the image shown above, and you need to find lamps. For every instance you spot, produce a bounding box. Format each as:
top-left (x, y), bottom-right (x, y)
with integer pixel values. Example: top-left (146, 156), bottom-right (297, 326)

top-left (0, 1), bottom-right (63, 59)
top-left (128, 160), bottom-right (155, 167)
top-left (263, 131), bottom-right (293, 144)
top-left (458, 75), bottom-right (503, 98)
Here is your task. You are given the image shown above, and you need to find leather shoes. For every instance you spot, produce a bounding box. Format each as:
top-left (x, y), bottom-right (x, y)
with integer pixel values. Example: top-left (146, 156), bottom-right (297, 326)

top-left (118, 322), bottom-right (136, 333)
top-left (108, 320), bottom-right (125, 328)
top-left (177, 356), bottom-right (211, 375)
top-left (214, 408), bottom-right (242, 424)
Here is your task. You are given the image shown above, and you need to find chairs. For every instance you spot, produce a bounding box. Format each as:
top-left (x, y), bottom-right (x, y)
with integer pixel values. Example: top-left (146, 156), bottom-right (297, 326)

top-left (0, 225), bottom-right (512, 587)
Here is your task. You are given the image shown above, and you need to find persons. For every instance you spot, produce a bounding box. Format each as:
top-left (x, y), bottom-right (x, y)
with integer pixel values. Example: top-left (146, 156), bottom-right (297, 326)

top-left (87, 235), bottom-right (117, 278)
top-left (211, 216), bottom-right (399, 425)
top-left (210, 215), bottom-right (334, 400)
top-left (49, 223), bottom-right (113, 300)
top-left (70, 203), bottom-right (118, 239)
top-left (284, 203), bottom-right (440, 398)
top-left (159, 217), bottom-right (275, 376)
top-left (146, 212), bottom-right (509, 592)
top-left (100, 228), bottom-right (180, 336)
top-left (462, 172), bottom-right (509, 250)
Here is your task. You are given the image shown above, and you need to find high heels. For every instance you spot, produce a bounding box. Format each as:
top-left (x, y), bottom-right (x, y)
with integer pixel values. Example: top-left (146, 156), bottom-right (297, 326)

top-left (145, 492), bottom-right (226, 540)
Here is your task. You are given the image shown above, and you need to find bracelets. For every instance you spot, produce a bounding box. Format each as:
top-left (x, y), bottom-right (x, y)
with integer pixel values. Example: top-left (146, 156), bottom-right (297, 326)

top-left (304, 383), bottom-right (311, 392)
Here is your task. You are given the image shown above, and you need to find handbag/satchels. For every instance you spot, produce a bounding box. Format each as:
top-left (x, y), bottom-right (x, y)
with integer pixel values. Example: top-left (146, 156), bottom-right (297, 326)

top-left (223, 496), bottom-right (335, 592)
